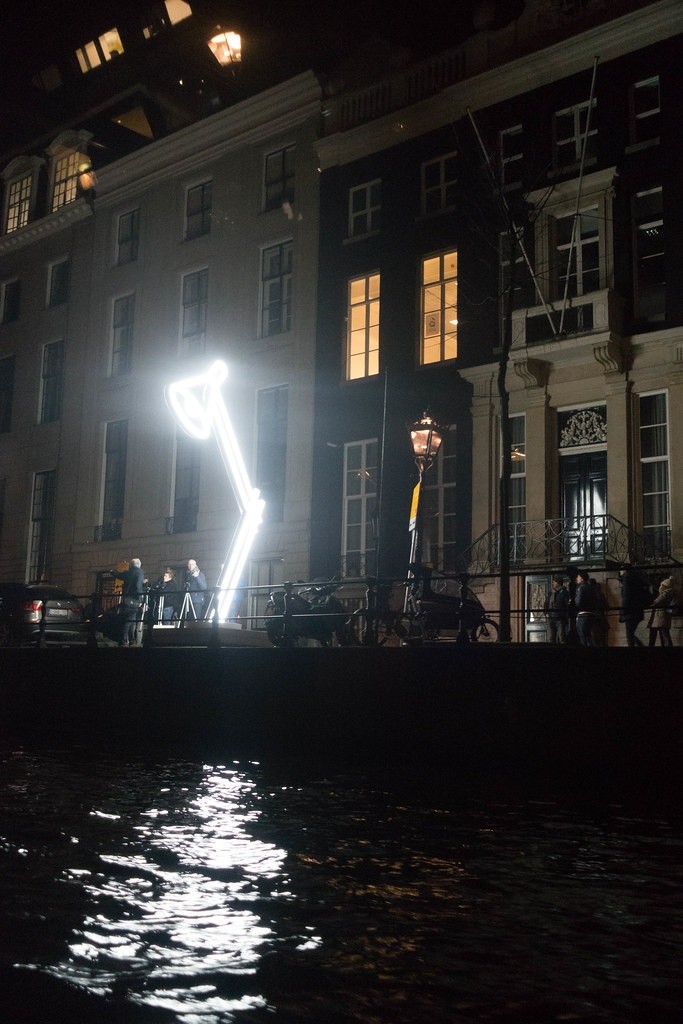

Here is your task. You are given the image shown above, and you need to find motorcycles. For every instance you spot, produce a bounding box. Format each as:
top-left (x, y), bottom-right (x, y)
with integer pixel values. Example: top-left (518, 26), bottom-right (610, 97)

top-left (266, 576), bottom-right (352, 647)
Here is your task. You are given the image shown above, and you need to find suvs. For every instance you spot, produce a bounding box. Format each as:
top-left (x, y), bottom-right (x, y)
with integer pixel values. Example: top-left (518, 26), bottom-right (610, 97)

top-left (1, 584), bottom-right (88, 641)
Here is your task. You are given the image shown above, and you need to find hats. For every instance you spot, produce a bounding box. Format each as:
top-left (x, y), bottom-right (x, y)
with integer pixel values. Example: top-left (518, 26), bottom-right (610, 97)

top-left (579, 570), bottom-right (589, 581)
top-left (552, 576), bottom-right (564, 586)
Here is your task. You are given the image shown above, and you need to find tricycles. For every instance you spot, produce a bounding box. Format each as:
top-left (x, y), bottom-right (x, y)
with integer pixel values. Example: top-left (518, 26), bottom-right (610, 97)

top-left (406, 562), bottom-right (499, 645)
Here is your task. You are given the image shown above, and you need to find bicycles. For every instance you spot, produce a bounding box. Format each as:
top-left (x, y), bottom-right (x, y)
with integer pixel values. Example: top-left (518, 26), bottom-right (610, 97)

top-left (347, 578), bottom-right (440, 646)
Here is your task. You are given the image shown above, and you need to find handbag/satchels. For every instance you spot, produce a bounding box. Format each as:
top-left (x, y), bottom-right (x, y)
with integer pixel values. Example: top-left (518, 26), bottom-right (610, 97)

top-left (122, 596), bottom-right (140, 613)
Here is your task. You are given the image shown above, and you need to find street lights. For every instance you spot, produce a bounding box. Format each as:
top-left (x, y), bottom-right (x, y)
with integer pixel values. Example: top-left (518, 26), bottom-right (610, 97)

top-left (399, 405), bottom-right (449, 647)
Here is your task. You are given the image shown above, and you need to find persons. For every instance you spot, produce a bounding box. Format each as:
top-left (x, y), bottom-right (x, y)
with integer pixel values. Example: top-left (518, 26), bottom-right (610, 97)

top-left (109, 558), bottom-right (144, 646)
top-left (543, 577), bottom-right (572, 644)
top-left (645, 576), bottom-right (677, 647)
top-left (183, 559), bottom-right (207, 623)
top-left (588, 578), bottom-right (610, 647)
top-left (574, 572), bottom-right (599, 646)
top-left (154, 571), bottom-right (177, 625)
top-left (618, 565), bottom-right (645, 647)
